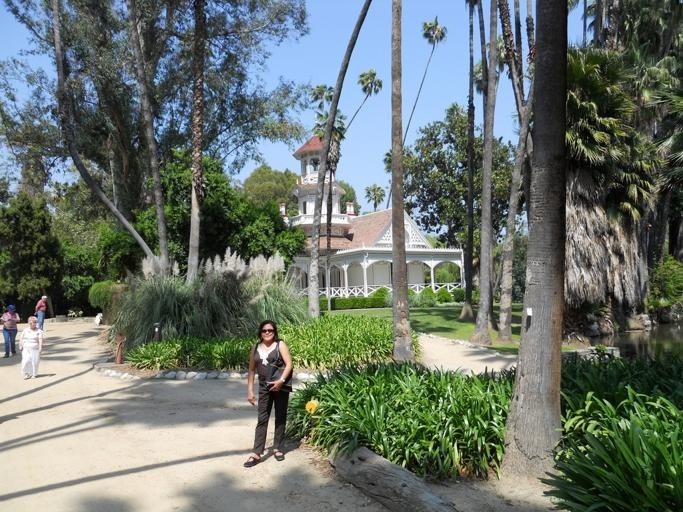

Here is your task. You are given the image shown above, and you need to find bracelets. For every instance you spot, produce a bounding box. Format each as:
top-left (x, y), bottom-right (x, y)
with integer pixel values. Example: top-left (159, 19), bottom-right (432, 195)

top-left (279, 375), bottom-right (287, 384)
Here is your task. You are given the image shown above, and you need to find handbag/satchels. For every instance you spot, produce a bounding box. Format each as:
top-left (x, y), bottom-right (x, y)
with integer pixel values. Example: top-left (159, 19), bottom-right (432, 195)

top-left (264, 358), bottom-right (293, 392)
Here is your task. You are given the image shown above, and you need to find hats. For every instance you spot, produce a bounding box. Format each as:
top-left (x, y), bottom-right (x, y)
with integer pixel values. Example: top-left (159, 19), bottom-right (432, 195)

top-left (9, 305), bottom-right (16, 311)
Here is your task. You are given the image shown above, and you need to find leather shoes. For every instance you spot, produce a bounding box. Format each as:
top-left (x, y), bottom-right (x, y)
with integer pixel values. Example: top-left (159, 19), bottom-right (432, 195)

top-left (244, 456), bottom-right (260, 467)
top-left (273, 448), bottom-right (284, 461)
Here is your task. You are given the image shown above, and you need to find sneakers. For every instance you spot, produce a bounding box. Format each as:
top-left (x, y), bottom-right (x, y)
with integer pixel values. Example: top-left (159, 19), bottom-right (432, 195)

top-left (24, 375), bottom-right (36, 379)
top-left (3, 354), bottom-right (9, 358)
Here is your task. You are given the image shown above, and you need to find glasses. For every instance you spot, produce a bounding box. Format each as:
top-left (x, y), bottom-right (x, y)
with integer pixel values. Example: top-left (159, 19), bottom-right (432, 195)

top-left (261, 329), bottom-right (274, 332)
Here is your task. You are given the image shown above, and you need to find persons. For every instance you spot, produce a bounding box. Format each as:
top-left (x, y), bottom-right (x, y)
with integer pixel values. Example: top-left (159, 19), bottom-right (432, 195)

top-left (242, 319), bottom-right (297, 467)
top-left (0, 304), bottom-right (20, 357)
top-left (17, 315), bottom-right (44, 380)
top-left (33, 295), bottom-right (48, 333)
